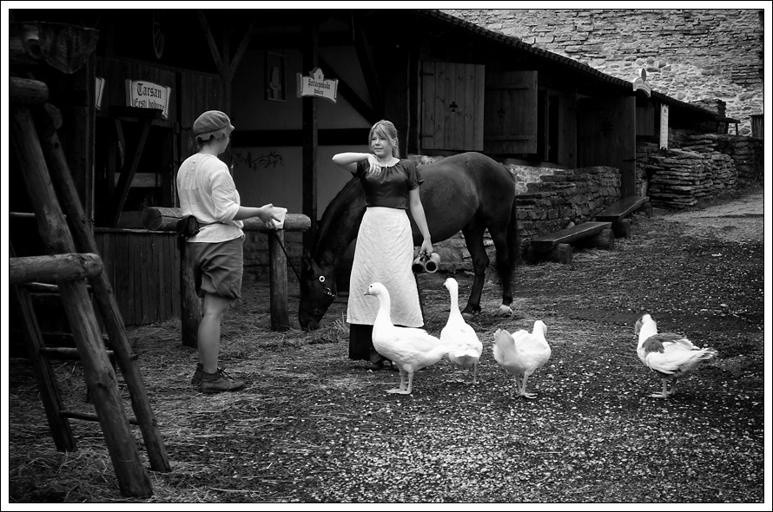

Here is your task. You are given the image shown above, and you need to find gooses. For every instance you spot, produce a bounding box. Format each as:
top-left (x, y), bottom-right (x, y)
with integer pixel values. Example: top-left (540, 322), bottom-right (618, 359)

top-left (491, 319), bottom-right (551, 399)
top-left (634, 313), bottom-right (720, 399)
top-left (363, 282), bottom-right (451, 394)
top-left (439, 277), bottom-right (483, 385)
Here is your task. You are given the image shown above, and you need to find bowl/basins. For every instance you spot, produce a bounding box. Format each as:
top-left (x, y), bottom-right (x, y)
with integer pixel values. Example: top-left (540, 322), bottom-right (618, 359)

top-left (266, 207), bottom-right (287, 230)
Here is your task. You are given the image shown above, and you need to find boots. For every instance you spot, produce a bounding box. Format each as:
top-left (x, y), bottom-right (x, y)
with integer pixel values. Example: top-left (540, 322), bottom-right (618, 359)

top-left (192, 363), bottom-right (244, 393)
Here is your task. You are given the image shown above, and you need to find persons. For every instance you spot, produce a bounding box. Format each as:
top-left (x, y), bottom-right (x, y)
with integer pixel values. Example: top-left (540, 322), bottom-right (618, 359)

top-left (175, 109), bottom-right (281, 395)
top-left (331, 120), bottom-right (435, 372)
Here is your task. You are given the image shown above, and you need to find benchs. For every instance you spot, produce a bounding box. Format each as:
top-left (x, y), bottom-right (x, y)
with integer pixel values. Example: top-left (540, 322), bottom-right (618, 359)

top-left (532, 196), bottom-right (653, 264)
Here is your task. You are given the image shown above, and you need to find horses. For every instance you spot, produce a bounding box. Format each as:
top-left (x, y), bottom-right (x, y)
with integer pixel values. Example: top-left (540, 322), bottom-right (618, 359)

top-left (298, 151), bottom-right (520, 332)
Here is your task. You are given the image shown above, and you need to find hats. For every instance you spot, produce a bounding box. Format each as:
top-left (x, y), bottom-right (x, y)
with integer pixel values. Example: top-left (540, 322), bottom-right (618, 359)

top-left (192, 111), bottom-right (235, 144)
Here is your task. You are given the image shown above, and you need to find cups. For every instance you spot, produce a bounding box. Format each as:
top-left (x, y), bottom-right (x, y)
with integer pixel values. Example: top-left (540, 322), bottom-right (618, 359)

top-left (411, 252), bottom-right (440, 273)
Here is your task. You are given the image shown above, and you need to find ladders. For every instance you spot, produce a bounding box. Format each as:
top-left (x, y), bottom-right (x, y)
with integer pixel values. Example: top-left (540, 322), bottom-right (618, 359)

top-left (10, 80), bottom-right (172, 473)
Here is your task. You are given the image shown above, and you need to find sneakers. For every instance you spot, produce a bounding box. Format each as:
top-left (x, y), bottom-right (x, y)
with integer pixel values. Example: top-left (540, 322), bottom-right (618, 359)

top-left (366, 360), bottom-right (384, 369)
